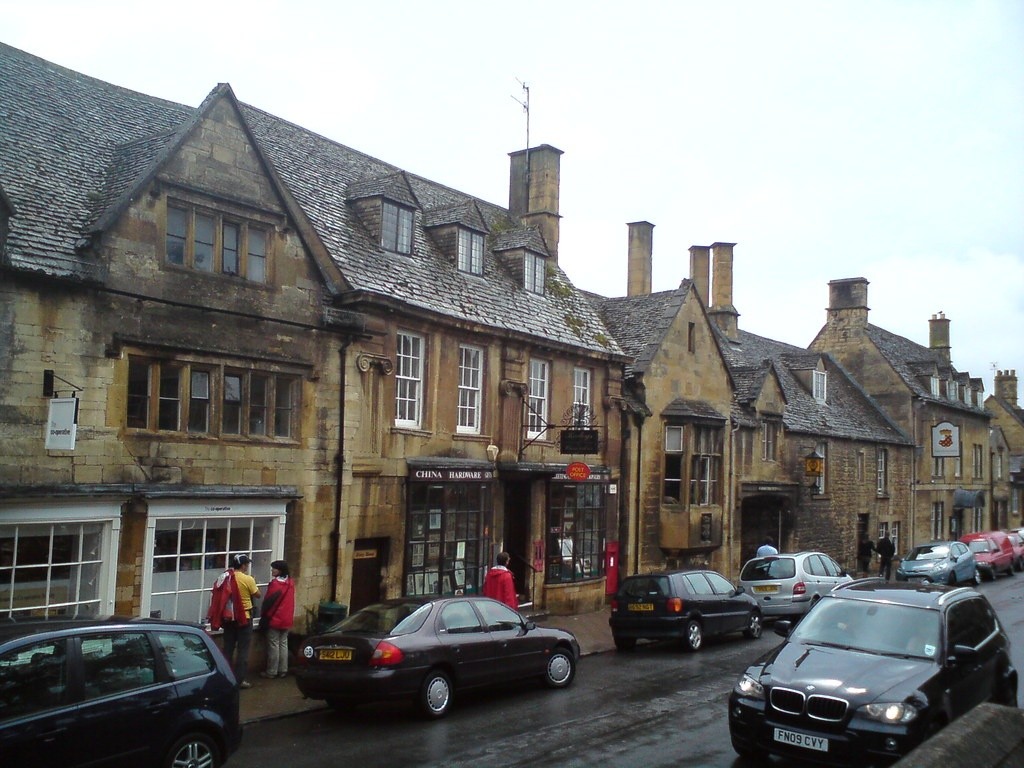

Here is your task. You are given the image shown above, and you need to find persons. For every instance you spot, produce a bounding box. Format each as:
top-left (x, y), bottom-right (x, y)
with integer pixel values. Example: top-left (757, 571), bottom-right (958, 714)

top-left (757, 536), bottom-right (778, 557)
top-left (482, 552), bottom-right (519, 612)
top-left (858, 533), bottom-right (877, 578)
top-left (222, 554), bottom-right (261, 688)
top-left (877, 531), bottom-right (895, 581)
top-left (262, 560), bottom-right (295, 680)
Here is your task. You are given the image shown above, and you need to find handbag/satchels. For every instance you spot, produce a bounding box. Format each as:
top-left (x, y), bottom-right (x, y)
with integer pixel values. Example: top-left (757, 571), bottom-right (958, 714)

top-left (258, 613), bottom-right (269, 628)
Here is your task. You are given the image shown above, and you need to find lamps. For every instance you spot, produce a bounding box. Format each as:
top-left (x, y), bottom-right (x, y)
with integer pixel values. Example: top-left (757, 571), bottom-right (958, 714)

top-left (801, 445), bottom-right (824, 494)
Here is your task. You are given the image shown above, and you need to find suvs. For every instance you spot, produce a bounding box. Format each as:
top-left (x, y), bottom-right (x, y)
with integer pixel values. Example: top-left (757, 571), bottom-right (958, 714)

top-left (729, 577), bottom-right (1018, 768)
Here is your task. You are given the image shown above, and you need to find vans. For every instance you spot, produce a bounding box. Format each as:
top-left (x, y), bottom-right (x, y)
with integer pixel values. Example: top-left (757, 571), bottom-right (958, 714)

top-left (959, 532), bottom-right (1016, 580)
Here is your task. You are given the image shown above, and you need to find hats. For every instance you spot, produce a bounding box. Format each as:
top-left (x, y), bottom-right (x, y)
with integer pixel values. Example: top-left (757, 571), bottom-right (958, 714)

top-left (233, 554), bottom-right (254, 568)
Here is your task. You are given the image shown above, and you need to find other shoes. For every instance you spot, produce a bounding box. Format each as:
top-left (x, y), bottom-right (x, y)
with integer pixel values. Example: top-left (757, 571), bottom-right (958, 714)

top-left (278, 672), bottom-right (286, 678)
top-left (260, 671), bottom-right (275, 679)
top-left (238, 680), bottom-right (252, 688)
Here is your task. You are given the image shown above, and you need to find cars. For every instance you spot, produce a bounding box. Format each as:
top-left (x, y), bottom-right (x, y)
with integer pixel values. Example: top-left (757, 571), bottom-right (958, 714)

top-left (0, 616), bottom-right (243, 768)
top-left (1007, 528), bottom-right (1024, 571)
top-left (608, 568), bottom-right (764, 650)
top-left (293, 597), bottom-right (580, 718)
top-left (738, 550), bottom-right (853, 616)
top-left (894, 541), bottom-right (982, 586)
top-left (549, 537), bottom-right (593, 577)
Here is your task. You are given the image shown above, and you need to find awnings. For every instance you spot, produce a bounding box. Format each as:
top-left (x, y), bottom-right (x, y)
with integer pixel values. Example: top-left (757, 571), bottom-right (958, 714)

top-left (952, 489), bottom-right (985, 509)
